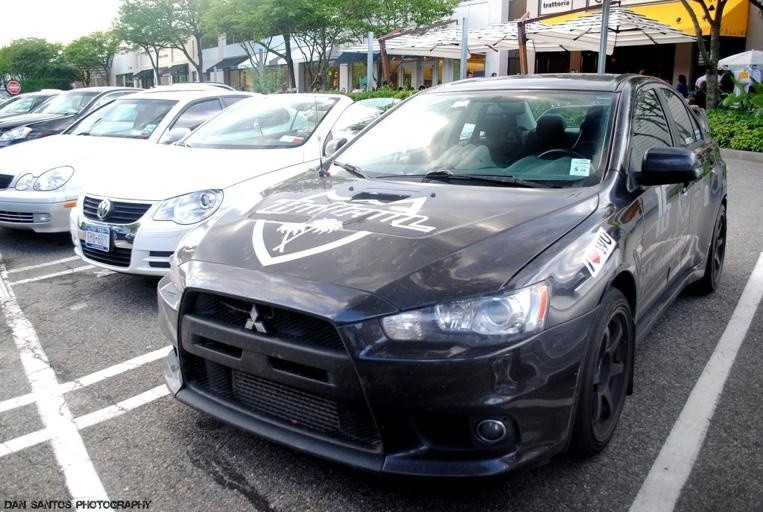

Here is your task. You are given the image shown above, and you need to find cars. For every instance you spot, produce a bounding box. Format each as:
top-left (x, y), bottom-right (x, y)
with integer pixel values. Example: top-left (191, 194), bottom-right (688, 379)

top-left (154, 73), bottom-right (730, 480)
top-left (0, 82), bottom-right (261, 241)
top-left (68, 92), bottom-right (402, 286)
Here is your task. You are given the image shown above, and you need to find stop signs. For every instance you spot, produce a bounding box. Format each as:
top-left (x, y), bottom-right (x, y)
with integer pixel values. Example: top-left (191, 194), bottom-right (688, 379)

top-left (6, 80), bottom-right (21, 94)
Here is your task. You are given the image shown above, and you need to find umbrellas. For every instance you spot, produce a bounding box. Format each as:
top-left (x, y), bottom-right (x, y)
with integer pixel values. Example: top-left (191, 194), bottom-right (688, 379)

top-left (717, 49), bottom-right (763, 69)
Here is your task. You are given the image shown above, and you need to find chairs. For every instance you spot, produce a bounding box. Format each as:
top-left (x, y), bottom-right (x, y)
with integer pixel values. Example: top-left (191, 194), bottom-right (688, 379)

top-left (468, 117), bottom-right (518, 169)
top-left (520, 114), bottom-right (578, 159)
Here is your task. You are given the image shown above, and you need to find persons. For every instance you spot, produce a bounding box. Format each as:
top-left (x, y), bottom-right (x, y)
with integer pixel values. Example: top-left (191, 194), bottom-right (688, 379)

top-left (467, 72), bottom-right (472, 78)
top-left (638, 66), bottom-right (763, 111)
top-left (280, 81), bottom-right (425, 92)
top-left (491, 73), bottom-right (497, 77)
top-left (570, 69), bottom-right (576, 73)
